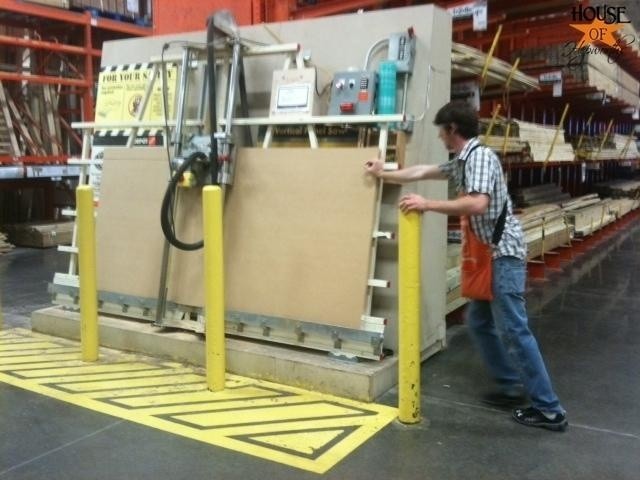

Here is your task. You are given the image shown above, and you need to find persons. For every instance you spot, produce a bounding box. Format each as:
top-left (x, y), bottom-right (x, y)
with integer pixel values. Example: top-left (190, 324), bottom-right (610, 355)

top-left (363, 100), bottom-right (568, 431)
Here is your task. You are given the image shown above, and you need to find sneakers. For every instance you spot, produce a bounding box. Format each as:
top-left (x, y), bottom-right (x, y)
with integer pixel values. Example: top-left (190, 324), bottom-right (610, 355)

top-left (512, 407), bottom-right (568, 431)
top-left (483, 392), bottom-right (525, 409)
